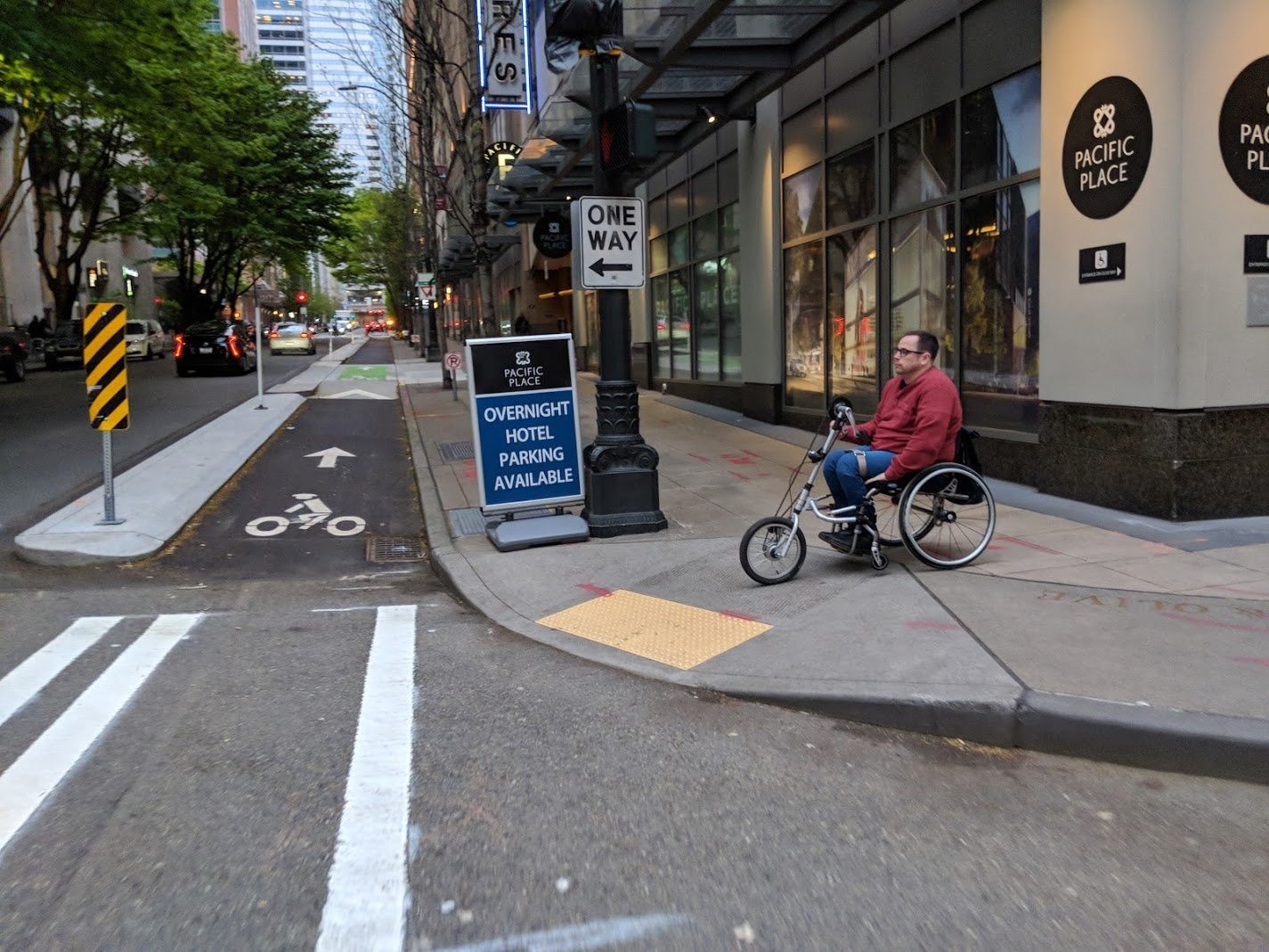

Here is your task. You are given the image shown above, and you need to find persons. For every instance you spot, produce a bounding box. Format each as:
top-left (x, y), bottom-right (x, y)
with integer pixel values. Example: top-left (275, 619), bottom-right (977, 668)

top-left (28, 315), bottom-right (53, 341)
top-left (818, 331), bottom-right (962, 556)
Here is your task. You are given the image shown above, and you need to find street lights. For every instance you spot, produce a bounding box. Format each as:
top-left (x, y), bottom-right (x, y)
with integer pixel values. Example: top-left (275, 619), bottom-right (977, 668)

top-left (338, 84), bottom-right (441, 363)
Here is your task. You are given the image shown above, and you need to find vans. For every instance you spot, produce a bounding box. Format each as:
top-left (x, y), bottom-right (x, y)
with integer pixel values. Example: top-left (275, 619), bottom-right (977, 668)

top-left (125, 318), bottom-right (166, 361)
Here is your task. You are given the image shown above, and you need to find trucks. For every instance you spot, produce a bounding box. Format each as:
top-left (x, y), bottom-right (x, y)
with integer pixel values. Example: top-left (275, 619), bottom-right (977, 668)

top-left (334, 309), bottom-right (354, 331)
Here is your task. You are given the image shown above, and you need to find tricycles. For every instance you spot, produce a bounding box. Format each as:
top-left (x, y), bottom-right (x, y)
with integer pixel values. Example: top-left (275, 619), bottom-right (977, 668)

top-left (738, 392), bottom-right (996, 586)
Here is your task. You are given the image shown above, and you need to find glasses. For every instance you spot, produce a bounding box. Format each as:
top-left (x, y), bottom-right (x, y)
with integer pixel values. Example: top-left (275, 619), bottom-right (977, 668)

top-left (893, 347), bottom-right (926, 357)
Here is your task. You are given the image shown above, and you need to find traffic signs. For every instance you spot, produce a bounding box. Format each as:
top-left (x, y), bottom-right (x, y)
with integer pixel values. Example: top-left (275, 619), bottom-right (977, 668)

top-left (580, 196), bottom-right (645, 288)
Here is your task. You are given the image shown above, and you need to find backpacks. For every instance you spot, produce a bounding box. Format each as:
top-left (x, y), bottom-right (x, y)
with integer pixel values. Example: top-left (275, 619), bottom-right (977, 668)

top-left (944, 425), bottom-right (983, 504)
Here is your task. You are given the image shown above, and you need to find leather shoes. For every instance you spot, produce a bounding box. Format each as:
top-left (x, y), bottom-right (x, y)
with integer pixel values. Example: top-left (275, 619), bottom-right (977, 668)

top-left (819, 528), bottom-right (881, 555)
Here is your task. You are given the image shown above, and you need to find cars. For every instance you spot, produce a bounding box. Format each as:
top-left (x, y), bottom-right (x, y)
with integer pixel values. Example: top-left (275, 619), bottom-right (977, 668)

top-left (174, 318), bottom-right (257, 376)
top-left (269, 321), bottom-right (318, 355)
top-left (364, 320), bottom-right (382, 332)
top-left (0, 331), bottom-right (46, 382)
top-left (44, 319), bottom-right (83, 370)
top-left (307, 322), bottom-right (336, 334)
